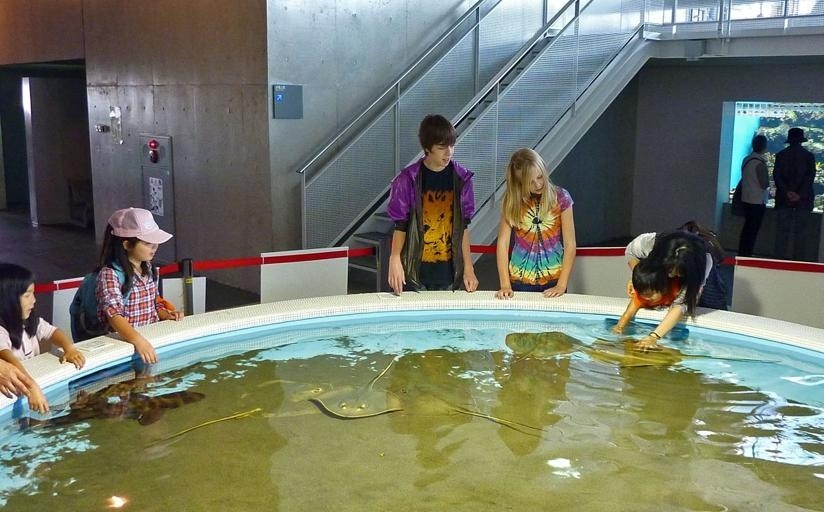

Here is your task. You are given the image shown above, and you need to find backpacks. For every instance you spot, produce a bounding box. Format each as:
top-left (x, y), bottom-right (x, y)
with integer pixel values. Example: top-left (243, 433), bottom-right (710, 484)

top-left (676, 221), bottom-right (724, 264)
top-left (69, 260), bottom-right (131, 343)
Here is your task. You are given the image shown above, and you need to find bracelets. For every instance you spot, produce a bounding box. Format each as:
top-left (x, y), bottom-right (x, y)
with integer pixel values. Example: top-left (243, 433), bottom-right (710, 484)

top-left (649, 332), bottom-right (661, 339)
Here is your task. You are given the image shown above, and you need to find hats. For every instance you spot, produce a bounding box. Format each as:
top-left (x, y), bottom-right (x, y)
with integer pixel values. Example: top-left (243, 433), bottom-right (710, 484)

top-left (107, 207), bottom-right (173, 244)
top-left (784, 128), bottom-right (808, 143)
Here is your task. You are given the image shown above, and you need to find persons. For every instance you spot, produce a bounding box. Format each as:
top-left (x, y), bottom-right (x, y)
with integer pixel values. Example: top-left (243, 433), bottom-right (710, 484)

top-left (737, 134), bottom-right (772, 256)
top-left (492, 145), bottom-right (578, 299)
top-left (772, 126), bottom-right (817, 260)
top-left (386, 110), bottom-right (481, 295)
top-left (612, 257), bottom-right (680, 336)
top-left (1, 261), bottom-right (86, 415)
top-left (0, 358), bottom-right (34, 397)
top-left (624, 219), bottom-right (736, 353)
top-left (91, 204), bottom-right (183, 364)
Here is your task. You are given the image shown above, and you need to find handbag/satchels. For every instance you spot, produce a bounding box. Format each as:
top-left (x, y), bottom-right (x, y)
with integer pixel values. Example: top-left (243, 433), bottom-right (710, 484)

top-left (731, 179), bottom-right (741, 216)
